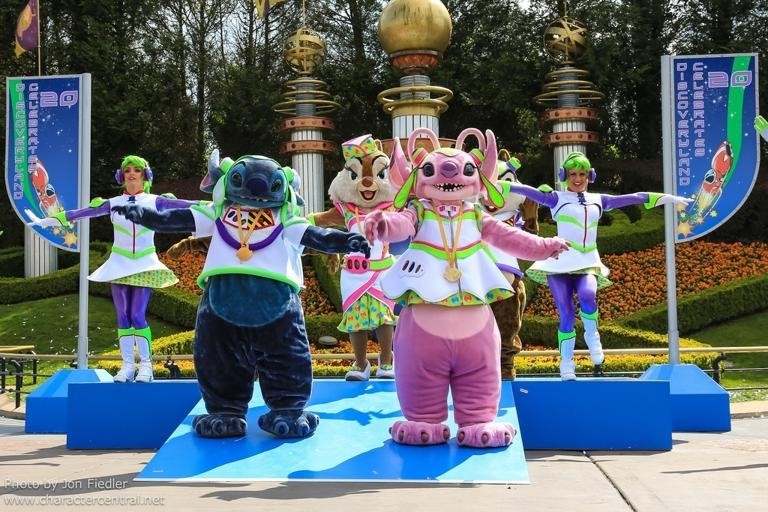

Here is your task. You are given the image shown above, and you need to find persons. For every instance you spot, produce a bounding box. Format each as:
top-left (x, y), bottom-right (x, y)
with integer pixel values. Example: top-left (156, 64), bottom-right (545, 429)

top-left (24, 155), bottom-right (218, 381)
top-left (498, 151), bottom-right (695, 381)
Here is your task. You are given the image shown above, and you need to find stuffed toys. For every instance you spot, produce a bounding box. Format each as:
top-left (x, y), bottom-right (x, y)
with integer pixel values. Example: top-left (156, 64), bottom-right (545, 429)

top-left (110, 149), bottom-right (371, 440)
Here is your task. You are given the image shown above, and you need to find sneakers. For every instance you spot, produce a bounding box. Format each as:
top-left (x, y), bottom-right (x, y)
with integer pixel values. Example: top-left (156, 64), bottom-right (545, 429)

top-left (561, 373), bottom-right (576, 380)
top-left (584, 334), bottom-right (603, 365)
top-left (114, 374), bottom-right (134, 382)
top-left (135, 375), bottom-right (154, 382)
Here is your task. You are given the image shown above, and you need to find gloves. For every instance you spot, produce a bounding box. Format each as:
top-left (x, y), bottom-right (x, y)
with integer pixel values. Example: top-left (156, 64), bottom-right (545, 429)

top-left (27, 211), bottom-right (68, 228)
top-left (644, 192), bottom-right (695, 210)
